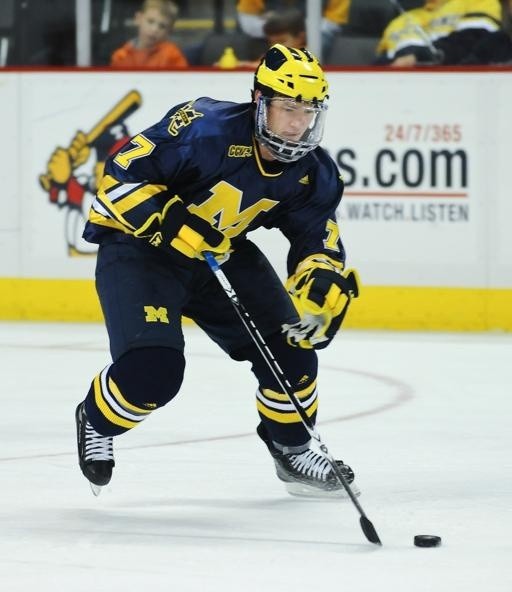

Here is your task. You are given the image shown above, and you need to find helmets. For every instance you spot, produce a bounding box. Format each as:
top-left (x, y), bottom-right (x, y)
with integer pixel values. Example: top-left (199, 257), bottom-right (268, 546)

top-left (250, 43), bottom-right (329, 164)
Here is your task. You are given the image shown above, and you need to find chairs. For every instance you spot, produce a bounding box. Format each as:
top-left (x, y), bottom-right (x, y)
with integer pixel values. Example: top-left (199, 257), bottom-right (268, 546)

top-left (98, 1), bottom-right (415, 66)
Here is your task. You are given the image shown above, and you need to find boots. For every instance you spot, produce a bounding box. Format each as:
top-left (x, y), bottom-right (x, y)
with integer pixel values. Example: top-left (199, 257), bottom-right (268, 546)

top-left (256, 420), bottom-right (354, 491)
top-left (75, 400), bottom-right (114, 486)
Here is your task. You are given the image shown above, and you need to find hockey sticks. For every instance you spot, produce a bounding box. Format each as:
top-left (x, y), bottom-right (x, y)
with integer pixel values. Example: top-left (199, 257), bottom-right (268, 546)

top-left (203, 252), bottom-right (380, 543)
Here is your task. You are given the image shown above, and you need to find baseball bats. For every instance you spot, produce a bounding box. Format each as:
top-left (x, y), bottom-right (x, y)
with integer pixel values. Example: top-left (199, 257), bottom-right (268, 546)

top-left (39, 90), bottom-right (142, 191)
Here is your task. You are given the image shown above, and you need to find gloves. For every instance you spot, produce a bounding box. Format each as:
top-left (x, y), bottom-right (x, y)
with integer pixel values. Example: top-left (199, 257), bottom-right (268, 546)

top-left (134, 197), bottom-right (231, 266)
top-left (283, 262), bottom-right (362, 351)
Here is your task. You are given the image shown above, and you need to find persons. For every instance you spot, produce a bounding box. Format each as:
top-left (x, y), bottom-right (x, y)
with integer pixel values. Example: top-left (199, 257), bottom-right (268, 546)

top-left (233, 1), bottom-right (355, 49)
top-left (261, 7), bottom-right (308, 51)
top-left (71, 40), bottom-right (363, 497)
top-left (108, 1), bottom-right (191, 68)
top-left (372, 0), bottom-right (512, 69)
